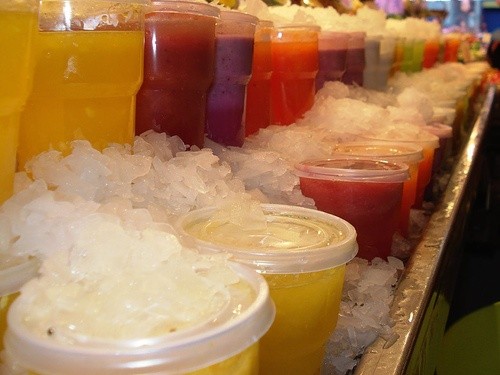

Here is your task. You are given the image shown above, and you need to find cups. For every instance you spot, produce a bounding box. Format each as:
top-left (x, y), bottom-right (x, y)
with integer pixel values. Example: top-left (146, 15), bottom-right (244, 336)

top-left (329, 141), bottom-right (425, 240)
top-left (176, 203), bottom-right (359, 375)
top-left (22, 0), bottom-right (147, 190)
top-left (318, 32), bottom-right (493, 187)
top-left (205, 10), bottom-right (258, 148)
top-left (0, 1), bottom-right (41, 204)
top-left (294, 159), bottom-right (411, 263)
top-left (135, 1), bottom-right (220, 151)
top-left (243, 19), bottom-right (273, 136)
top-left (7, 260), bottom-right (277, 375)
top-left (272, 25), bottom-right (322, 127)
